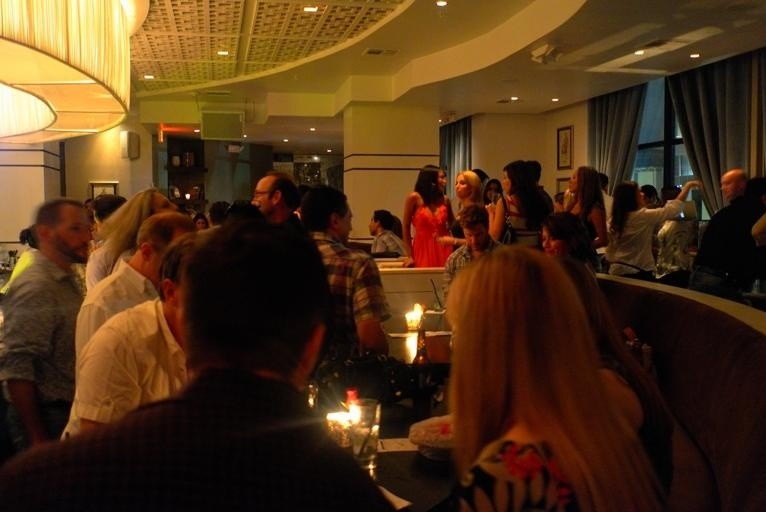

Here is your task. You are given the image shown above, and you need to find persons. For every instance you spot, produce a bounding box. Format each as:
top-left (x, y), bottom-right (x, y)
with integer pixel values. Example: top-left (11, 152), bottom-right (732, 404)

top-left (2, 157), bottom-right (765, 512)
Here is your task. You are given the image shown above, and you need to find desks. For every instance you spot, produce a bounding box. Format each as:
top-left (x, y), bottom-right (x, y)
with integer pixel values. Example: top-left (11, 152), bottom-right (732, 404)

top-left (320, 329), bottom-right (452, 510)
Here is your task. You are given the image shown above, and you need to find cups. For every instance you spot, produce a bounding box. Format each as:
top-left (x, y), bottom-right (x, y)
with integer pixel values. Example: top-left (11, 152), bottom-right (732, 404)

top-left (350, 399), bottom-right (381, 470)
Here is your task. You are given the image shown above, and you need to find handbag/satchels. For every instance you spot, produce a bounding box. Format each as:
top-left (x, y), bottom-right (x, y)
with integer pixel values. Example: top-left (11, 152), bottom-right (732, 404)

top-left (500, 196), bottom-right (516, 244)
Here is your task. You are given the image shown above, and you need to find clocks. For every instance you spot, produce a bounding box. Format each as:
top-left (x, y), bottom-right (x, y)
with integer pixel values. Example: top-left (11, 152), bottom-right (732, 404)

top-left (87, 181), bottom-right (119, 200)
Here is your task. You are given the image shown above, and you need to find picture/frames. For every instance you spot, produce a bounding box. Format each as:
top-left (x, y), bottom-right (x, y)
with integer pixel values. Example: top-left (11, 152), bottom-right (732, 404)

top-left (557, 125), bottom-right (573, 169)
top-left (556, 177), bottom-right (569, 194)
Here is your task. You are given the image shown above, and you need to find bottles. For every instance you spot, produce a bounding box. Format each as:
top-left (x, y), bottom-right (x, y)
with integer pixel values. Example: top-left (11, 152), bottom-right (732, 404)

top-left (413, 329), bottom-right (431, 406)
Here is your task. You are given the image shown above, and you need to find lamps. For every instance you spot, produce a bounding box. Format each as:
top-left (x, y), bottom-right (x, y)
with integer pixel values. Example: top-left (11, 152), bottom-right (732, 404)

top-left (0, 0), bottom-right (150, 145)
